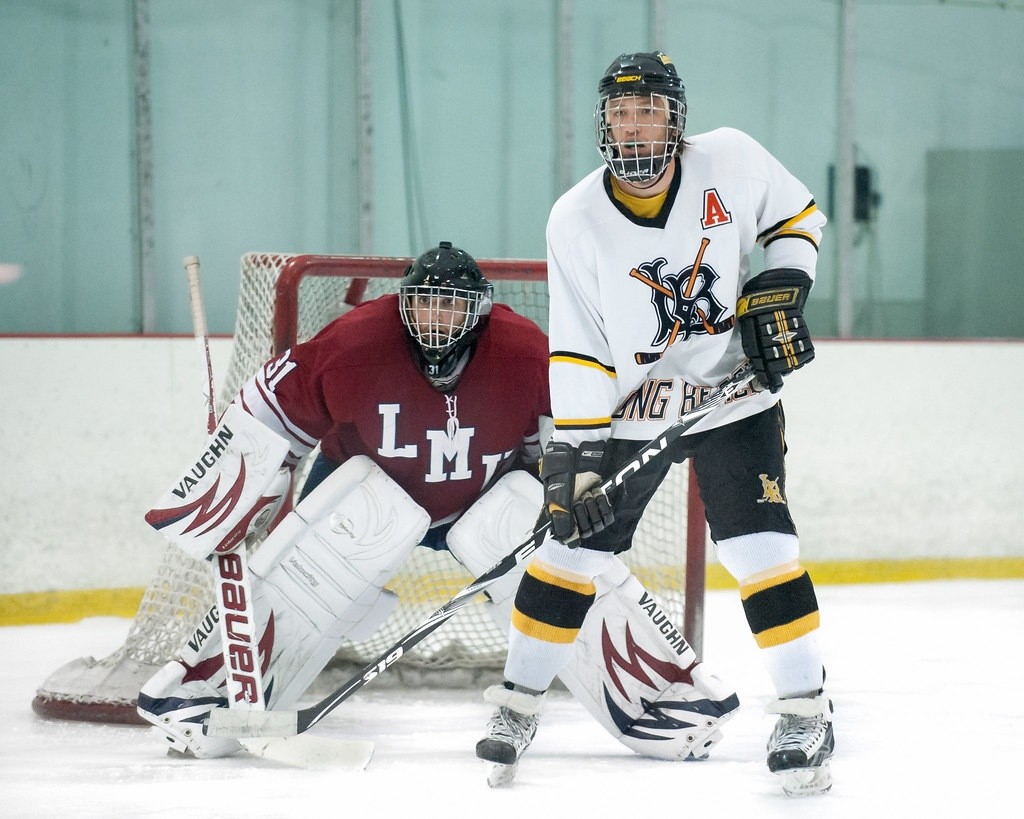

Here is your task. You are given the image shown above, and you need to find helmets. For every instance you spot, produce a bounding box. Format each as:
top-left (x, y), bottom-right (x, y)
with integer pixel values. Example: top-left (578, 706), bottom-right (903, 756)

top-left (594, 51), bottom-right (687, 184)
top-left (397, 242), bottom-right (494, 379)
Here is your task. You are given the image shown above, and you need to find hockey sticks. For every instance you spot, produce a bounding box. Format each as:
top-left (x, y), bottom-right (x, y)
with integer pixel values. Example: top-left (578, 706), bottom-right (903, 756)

top-left (182, 255), bottom-right (375, 772)
top-left (202, 357), bottom-right (758, 738)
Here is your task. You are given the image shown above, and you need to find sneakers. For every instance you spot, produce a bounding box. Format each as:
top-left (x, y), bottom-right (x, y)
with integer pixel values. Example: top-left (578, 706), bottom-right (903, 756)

top-left (475, 679), bottom-right (547, 785)
top-left (765, 663), bottom-right (835, 796)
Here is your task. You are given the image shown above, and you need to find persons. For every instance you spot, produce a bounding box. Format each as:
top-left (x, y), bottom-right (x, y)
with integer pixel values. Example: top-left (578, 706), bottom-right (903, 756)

top-left (131, 242), bottom-right (740, 765)
top-left (473, 50), bottom-right (839, 798)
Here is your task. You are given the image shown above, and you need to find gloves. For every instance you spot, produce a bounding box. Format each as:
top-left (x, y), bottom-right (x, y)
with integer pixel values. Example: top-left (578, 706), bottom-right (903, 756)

top-left (539, 438), bottom-right (616, 549)
top-left (734, 268), bottom-right (815, 393)
